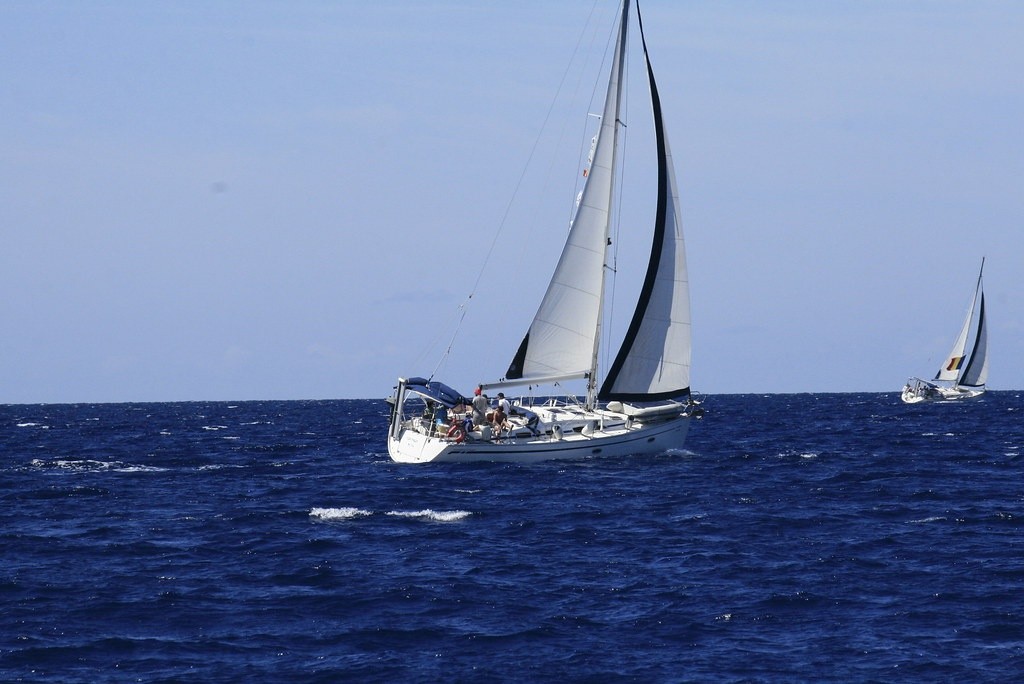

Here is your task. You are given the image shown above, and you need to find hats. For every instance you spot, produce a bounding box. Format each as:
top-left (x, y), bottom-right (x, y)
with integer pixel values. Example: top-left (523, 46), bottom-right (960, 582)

top-left (474, 388), bottom-right (481, 395)
top-left (498, 393), bottom-right (504, 398)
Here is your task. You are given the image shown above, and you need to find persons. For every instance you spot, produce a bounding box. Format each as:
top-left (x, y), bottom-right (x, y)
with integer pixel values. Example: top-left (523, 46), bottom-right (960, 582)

top-left (461, 388), bottom-right (511, 434)
top-left (903, 381), bottom-right (928, 396)
top-left (423, 401), bottom-right (455, 429)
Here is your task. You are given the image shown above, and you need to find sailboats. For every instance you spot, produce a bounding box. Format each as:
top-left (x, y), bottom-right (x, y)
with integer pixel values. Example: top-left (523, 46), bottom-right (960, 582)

top-left (384, 1), bottom-right (708, 464)
top-left (899, 256), bottom-right (991, 406)
